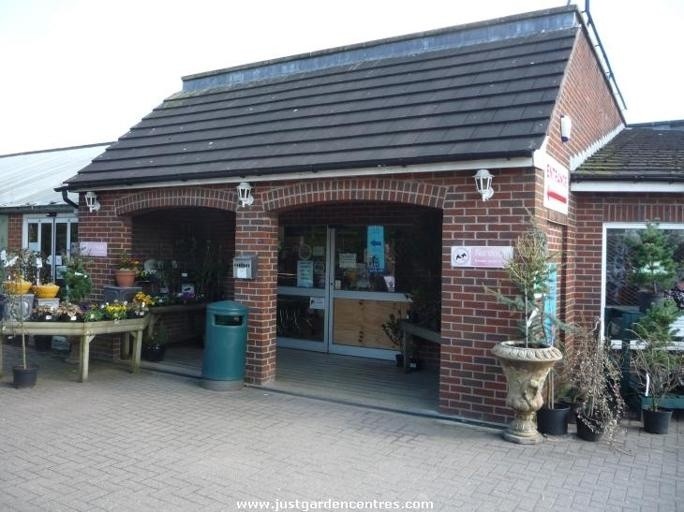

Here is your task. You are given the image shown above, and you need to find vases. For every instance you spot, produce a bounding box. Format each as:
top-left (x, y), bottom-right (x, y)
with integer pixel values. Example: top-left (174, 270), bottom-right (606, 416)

top-left (403, 283), bottom-right (440, 331)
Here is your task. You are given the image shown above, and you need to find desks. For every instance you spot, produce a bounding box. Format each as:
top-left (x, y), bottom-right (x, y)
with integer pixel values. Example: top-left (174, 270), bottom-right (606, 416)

top-left (142, 304), bottom-right (205, 360)
top-left (0, 314), bottom-right (151, 384)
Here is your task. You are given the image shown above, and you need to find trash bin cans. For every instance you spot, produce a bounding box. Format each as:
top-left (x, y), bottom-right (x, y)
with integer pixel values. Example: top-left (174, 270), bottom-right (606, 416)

top-left (200, 300), bottom-right (249, 392)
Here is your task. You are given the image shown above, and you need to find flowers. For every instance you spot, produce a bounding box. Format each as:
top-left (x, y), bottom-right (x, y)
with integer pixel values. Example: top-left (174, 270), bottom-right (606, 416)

top-left (1, 249), bottom-right (214, 322)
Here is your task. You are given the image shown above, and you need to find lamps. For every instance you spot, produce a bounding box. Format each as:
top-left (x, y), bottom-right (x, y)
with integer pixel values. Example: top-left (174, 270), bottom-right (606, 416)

top-left (471, 169), bottom-right (495, 203)
top-left (235, 182), bottom-right (255, 209)
top-left (84, 191), bottom-right (99, 213)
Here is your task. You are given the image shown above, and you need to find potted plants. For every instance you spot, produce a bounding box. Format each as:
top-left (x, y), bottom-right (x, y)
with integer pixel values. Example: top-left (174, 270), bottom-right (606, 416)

top-left (142, 318), bottom-right (166, 363)
top-left (563, 315), bottom-right (629, 443)
top-left (535, 264), bottom-right (573, 438)
top-left (380, 306), bottom-right (405, 368)
top-left (623, 216), bottom-right (681, 316)
top-left (0, 249), bottom-right (45, 389)
top-left (621, 321), bottom-right (684, 436)
top-left (487, 217), bottom-right (568, 447)
